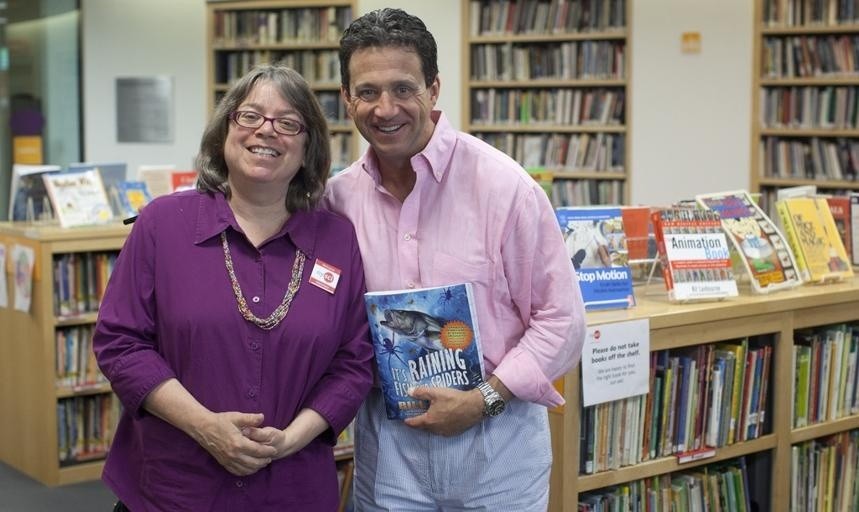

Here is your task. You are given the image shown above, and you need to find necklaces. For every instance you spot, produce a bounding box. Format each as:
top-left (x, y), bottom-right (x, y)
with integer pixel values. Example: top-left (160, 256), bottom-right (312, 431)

top-left (220, 228), bottom-right (306, 331)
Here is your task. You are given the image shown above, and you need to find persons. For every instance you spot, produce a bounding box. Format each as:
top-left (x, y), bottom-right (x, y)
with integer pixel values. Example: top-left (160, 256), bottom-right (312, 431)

top-left (91, 65), bottom-right (375, 510)
top-left (326, 26), bottom-right (588, 512)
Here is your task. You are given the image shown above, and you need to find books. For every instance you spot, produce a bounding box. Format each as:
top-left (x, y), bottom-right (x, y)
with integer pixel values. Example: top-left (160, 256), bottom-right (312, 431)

top-left (753, 0), bottom-right (858, 195)
top-left (694, 189), bottom-right (803, 294)
top-left (776, 195), bottom-right (856, 283)
top-left (556, 209), bottom-right (635, 310)
top-left (52, 250), bottom-right (119, 318)
top-left (579, 337), bottom-right (773, 477)
top-left (58, 391), bottom-right (125, 468)
top-left (459, 0), bottom-right (627, 209)
top-left (8, 165), bottom-right (199, 227)
top-left (55, 323), bottom-right (111, 387)
top-left (791, 428), bottom-right (859, 512)
top-left (793, 324), bottom-right (859, 429)
top-left (207, 1), bottom-right (360, 173)
top-left (577, 449), bottom-right (773, 512)
top-left (363, 284), bottom-right (484, 419)
top-left (655, 208), bottom-right (738, 300)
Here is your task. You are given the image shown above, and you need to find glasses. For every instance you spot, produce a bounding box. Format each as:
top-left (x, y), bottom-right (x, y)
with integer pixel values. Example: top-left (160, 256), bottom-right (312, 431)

top-left (227, 110), bottom-right (310, 138)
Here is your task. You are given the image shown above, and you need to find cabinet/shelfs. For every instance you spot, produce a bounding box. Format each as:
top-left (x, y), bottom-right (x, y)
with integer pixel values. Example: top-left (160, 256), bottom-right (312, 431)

top-left (341, 262), bottom-right (858, 511)
top-left (459, 1), bottom-right (631, 209)
top-left (752, 0), bottom-right (859, 215)
top-left (206, 0), bottom-right (358, 179)
top-left (1, 219), bottom-right (136, 490)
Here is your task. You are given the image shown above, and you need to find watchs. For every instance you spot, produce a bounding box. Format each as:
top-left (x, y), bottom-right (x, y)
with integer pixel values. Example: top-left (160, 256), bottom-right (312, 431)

top-left (476, 380), bottom-right (505, 418)
